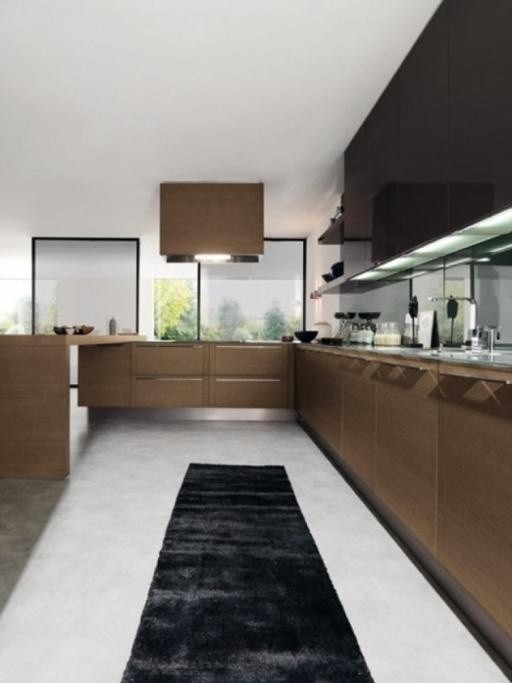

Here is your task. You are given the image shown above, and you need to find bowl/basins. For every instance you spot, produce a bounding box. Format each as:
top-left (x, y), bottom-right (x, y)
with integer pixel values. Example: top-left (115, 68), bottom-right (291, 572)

top-left (55, 325), bottom-right (94, 334)
top-left (66, 328), bottom-right (75, 334)
top-left (295, 330), bottom-right (318, 342)
top-left (321, 261), bottom-right (344, 282)
top-left (321, 336), bottom-right (331, 344)
top-left (332, 338), bottom-right (343, 344)
top-left (282, 335), bottom-right (293, 341)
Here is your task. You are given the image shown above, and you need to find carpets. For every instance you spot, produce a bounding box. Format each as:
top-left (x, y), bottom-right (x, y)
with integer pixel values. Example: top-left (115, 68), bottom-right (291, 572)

top-left (120, 464), bottom-right (376, 683)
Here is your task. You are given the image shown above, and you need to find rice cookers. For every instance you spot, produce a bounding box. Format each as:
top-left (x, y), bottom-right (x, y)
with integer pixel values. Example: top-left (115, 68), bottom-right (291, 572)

top-left (314, 321), bottom-right (333, 341)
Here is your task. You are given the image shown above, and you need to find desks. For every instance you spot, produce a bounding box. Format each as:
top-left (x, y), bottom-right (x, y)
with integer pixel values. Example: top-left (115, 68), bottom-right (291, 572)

top-left (0, 334), bottom-right (147, 480)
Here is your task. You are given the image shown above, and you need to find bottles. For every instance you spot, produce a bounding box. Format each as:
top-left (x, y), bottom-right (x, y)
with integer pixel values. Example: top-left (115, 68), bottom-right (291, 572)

top-left (109, 317), bottom-right (117, 335)
top-left (373, 321), bottom-right (402, 347)
top-left (350, 325), bottom-right (372, 343)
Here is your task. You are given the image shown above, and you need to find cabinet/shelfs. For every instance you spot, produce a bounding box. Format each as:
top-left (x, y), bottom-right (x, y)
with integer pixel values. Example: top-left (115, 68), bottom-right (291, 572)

top-left (375, 360), bottom-right (437, 558)
top-left (310, 215), bottom-right (343, 301)
top-left (295, 347), bottom-right (340, 457)
top-left (437, 361), bottom-right (511, 621)
top-left (341, 353), bottom-right (375, 494)
top-left (132, 343), bottom-right (295, 406)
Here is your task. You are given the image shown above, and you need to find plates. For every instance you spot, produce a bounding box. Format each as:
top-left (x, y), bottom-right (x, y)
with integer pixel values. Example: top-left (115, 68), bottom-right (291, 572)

top-left (119, 333), bottom-right (136, 335)
top-left (122, 328), bottom-right (131, 333)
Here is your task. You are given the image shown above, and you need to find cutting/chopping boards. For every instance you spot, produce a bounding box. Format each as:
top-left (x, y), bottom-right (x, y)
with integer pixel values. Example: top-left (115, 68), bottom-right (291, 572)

top-left (403, 309), bottom-right (435, 349)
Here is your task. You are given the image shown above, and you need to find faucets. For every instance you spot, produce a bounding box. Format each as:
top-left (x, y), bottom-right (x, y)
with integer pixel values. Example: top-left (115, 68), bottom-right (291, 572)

top-left (428, 296), bottom-right (477, 346)
